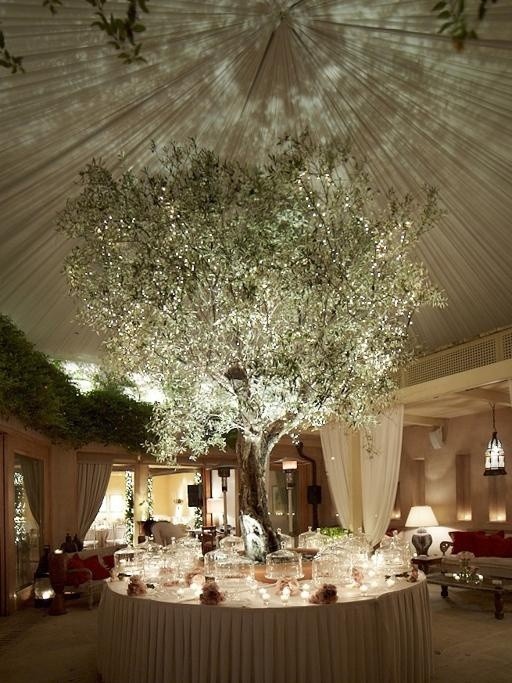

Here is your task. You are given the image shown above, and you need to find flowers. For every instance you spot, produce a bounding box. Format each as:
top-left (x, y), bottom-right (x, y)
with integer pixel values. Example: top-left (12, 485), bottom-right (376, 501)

top-left (456, 551), bottom-right (475, 559)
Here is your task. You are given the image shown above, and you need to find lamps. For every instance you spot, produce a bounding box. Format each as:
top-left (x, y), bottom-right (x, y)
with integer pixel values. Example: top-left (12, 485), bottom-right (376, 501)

top-left (483, 400), bottom-right (506, 476)
top-left (405, 506), bottom-right (437, 557)
top-left (218, 468), bottom-right (230, 491)
top-left (282, 461), bottom-right (297, 490)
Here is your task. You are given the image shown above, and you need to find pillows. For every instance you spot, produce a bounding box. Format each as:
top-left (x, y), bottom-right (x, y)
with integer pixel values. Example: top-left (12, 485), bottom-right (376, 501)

top-left (69, 552), bottom-right (114, 586)
top-left (448, 531), bottom-right (511, 557)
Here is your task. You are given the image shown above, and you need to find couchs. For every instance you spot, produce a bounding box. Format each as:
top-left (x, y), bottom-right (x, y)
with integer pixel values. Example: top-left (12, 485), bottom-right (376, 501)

top-left (439, 530), bottom-right (511, 577)
top-left (64, 546), bottom-right (116, 610)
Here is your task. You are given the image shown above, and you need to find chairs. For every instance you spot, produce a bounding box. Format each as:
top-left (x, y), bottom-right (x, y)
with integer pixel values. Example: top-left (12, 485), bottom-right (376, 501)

top-left (81, 524), bottom-right (128, 552)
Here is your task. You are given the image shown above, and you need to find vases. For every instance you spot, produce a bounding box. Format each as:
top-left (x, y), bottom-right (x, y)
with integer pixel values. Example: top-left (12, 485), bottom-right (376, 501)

top-left (460, 558), bottom-right (471, 577)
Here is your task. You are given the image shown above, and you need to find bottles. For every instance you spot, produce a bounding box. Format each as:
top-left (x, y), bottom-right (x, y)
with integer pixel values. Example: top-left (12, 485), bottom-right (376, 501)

top-left (113, 526), bottom-right (410, 606)
top-left (74, 533), bottom-right (78, 540)
top-left (66, 532), bottom-right (72, 543)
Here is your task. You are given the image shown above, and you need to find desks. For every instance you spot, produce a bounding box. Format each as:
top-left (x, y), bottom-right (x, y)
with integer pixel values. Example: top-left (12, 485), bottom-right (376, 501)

top-left (410, 557), bottom-right (442, 573)
